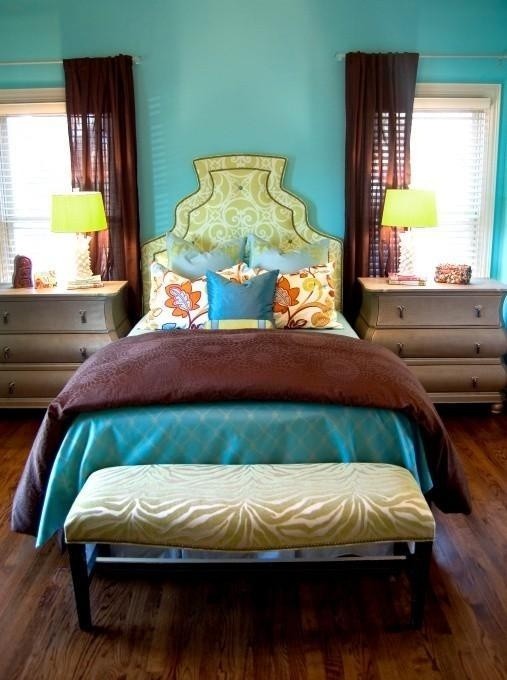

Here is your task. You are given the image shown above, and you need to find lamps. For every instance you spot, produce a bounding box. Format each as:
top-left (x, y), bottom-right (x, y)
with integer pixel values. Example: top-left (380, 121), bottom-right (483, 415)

top-left (381, 188), bottom-right (439, 275)
top-left (49, 190), bottom-right (108, 278)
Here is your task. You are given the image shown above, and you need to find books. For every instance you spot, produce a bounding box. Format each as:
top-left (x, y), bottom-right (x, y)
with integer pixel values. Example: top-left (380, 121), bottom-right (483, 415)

top-left (388, 273), bottom-right (427, 281)
top-left (67, 275), bottom-right (104, 290)
top-left (388, 276), bottom-right (427, 286)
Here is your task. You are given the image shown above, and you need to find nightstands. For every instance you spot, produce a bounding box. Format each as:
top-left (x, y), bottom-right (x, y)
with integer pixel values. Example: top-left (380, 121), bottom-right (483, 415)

top-left (356, 276), bottom-right (507, 414)
top-left (0, 279), bottom-right (133, 418)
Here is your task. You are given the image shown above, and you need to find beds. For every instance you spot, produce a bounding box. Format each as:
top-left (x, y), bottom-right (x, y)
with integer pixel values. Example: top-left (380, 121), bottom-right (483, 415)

top-left (10, 152), bottom-right (472, 563)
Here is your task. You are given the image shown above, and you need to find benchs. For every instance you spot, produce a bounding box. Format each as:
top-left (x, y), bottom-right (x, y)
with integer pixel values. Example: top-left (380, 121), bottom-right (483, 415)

top-left (62, 463), bottom-right (438, 630)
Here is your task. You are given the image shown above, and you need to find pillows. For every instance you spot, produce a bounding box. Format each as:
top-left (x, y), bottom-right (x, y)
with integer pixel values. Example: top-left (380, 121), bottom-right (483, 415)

top-left (254, 261), bottom-right (337, 330)
top-left (204, 268), bottom-right (280, 330)
top-left (135, 261), bottom-right (256, 331)
